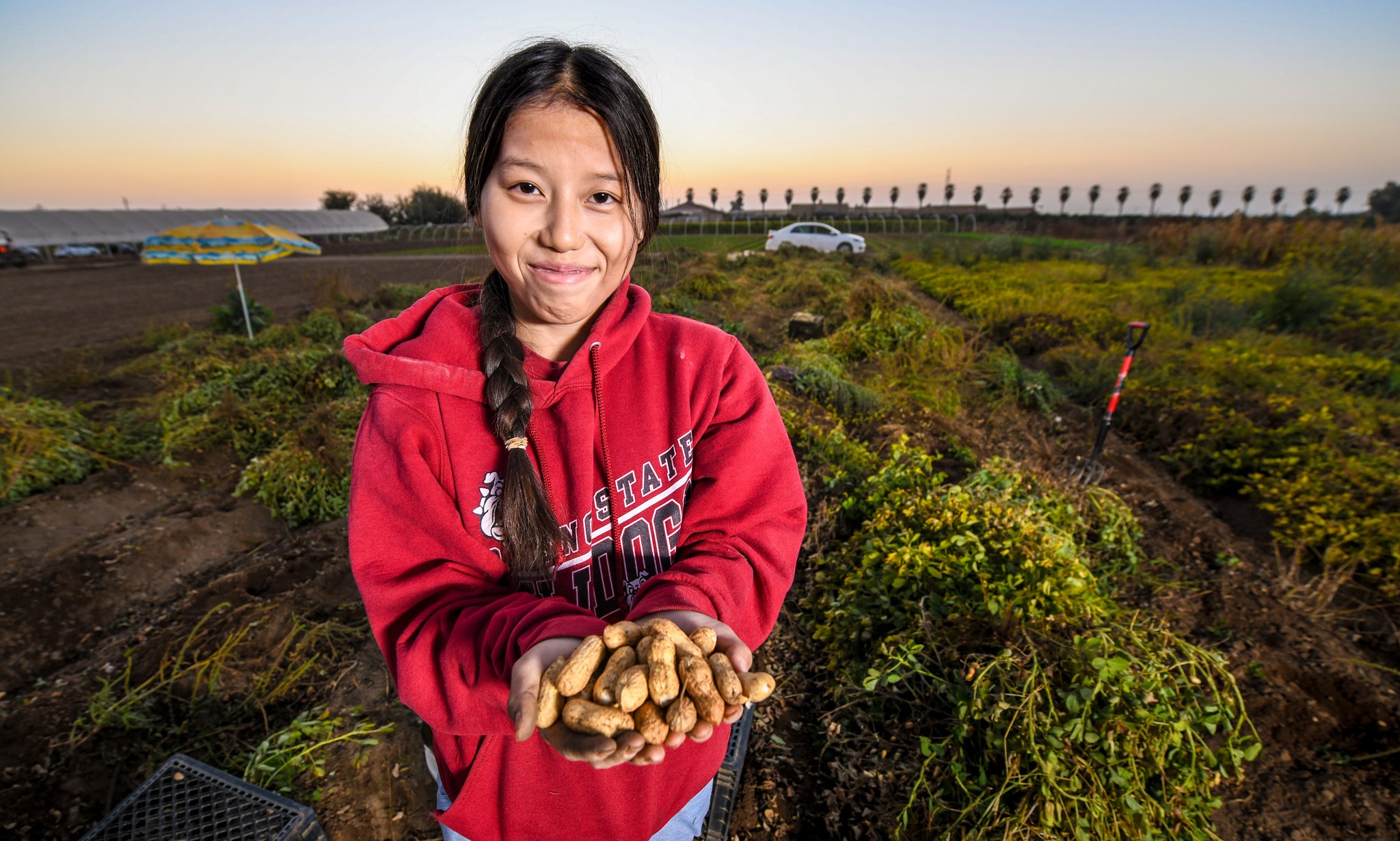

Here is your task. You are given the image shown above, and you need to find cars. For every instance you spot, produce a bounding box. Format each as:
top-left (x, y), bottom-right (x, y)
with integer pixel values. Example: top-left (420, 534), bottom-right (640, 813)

top-left (764, 223), bottom-right (867, 257)
top-left (0, 229), bottom-right (139, 264)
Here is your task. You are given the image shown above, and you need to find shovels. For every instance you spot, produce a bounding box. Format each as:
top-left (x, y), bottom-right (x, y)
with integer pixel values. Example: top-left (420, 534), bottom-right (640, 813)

top-left (1068, 319), bottom-right (1152, 488)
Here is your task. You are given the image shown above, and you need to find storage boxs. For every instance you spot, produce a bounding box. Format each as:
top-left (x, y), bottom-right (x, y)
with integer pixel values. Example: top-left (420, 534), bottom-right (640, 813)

top-left (77, 754), bottom-right (331, 841)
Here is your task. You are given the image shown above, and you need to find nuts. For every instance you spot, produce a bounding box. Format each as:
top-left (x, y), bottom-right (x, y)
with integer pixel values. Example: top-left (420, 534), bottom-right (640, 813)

top-left (532, 618), bottom-right (775, 747)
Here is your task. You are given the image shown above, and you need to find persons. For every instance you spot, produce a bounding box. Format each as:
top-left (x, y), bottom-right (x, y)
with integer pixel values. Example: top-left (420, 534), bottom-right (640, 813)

top-left (336, 35), bottom-right (809, 841)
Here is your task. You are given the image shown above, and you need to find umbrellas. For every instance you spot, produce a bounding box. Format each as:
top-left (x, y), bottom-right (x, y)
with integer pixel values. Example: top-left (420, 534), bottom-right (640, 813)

top-left (139, 212), bottom-right (323, 341)
